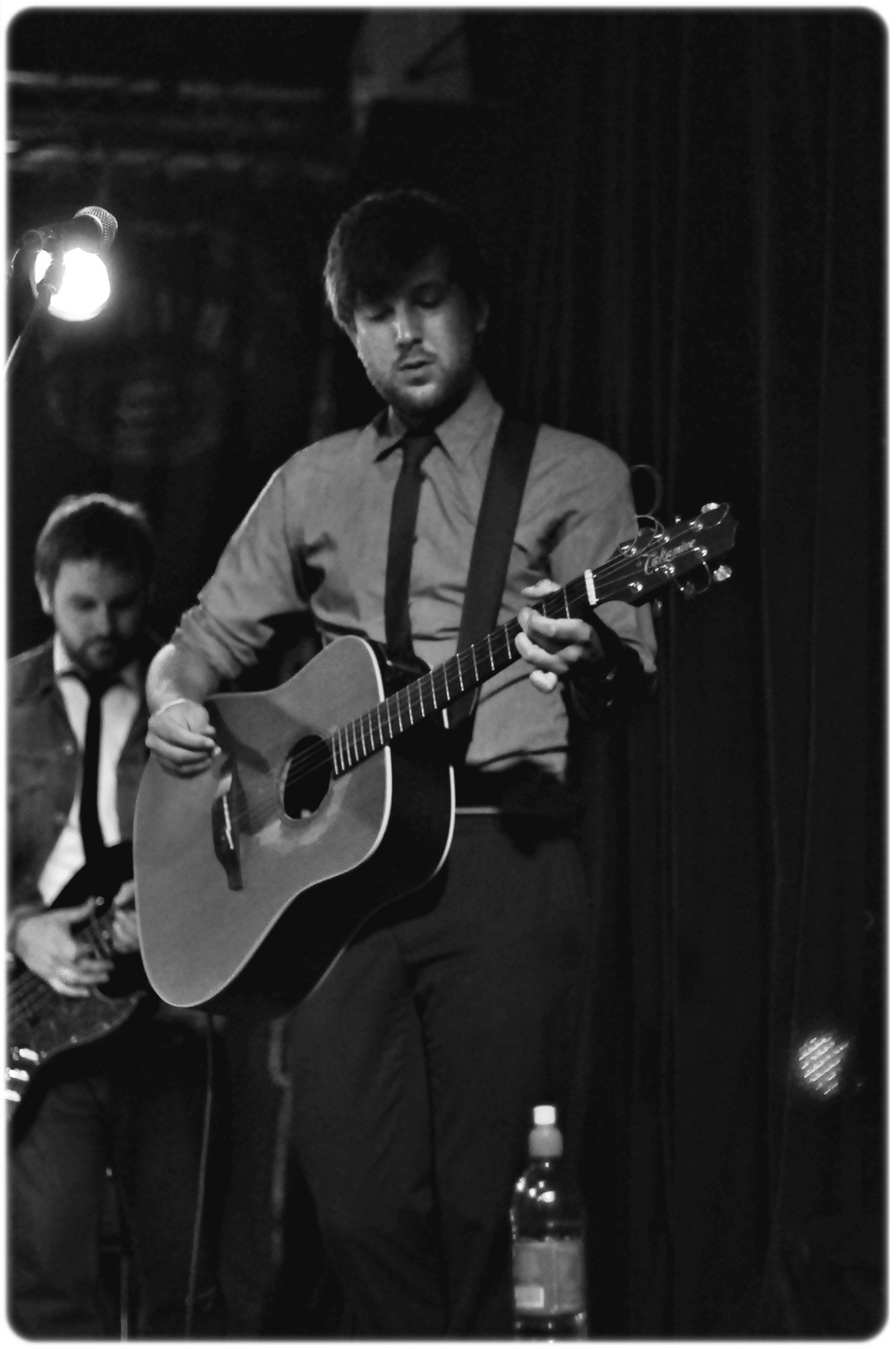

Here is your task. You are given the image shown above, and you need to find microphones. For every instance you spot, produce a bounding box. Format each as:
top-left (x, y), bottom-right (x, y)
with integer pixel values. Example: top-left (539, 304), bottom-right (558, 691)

top-left (21, 205), bottom-right (119, 255)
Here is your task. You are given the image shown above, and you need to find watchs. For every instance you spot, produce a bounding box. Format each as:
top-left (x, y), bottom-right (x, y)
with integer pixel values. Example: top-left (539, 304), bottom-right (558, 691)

top-left (587, 654), bottom-right (633, 691)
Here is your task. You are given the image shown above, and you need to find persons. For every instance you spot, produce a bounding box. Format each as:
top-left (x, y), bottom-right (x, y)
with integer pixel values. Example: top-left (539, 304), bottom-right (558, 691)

top-left (140, 189), bottom-right (658, 1340)
top-left (7, 491), bottom-right (242, 1339)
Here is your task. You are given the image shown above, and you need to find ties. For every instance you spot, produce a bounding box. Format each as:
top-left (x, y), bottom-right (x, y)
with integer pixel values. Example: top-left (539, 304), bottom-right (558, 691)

top-left (73, 672), bottom-right (123, 861)
top-left (384, 431), bottom-right (440, 659)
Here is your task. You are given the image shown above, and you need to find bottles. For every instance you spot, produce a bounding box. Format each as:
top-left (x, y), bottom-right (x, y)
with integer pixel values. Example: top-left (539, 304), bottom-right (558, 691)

top-left (507, 1105), bottom-right (591, 1341)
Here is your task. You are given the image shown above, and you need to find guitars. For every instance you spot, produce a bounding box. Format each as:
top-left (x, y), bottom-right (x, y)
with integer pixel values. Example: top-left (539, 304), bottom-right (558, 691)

top-left (132, 502), bottom-right (743, 1020)
top-left (6, 904), bottom-right (150, 1133)
